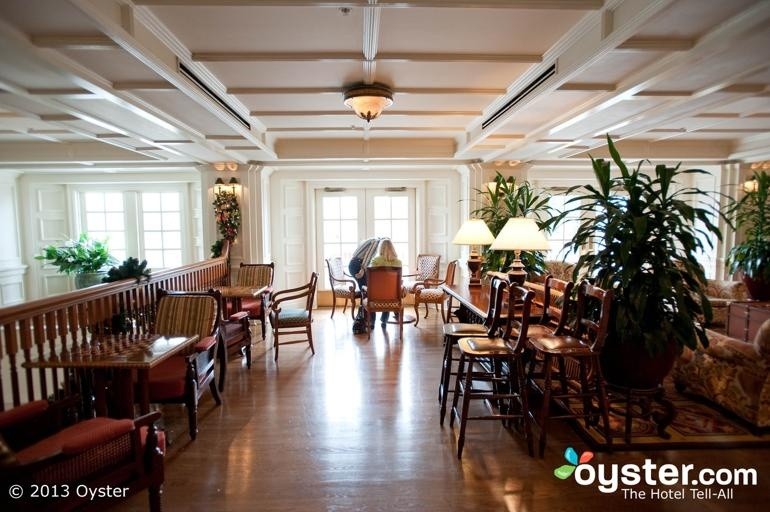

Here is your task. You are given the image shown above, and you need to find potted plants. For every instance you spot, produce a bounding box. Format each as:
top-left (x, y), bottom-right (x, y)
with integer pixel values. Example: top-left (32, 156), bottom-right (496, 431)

top-left (33, 230), bottom-right (120, 289)
top-left (724, 167), bottom-right (770, 301)
top-left (550, 131), bottom-right (737, 390)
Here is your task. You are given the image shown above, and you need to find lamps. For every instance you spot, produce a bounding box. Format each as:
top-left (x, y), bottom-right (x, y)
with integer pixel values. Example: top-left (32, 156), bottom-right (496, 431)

top-left (741, 176), bottom-right (759, 193)
top-left (488, 175), bottom-right (518, 197)
top-left (343, 85), bottom-right (394, 122)
top-left (452, 219), bottom-right (496, 287)
top-left (215, 177), bottom-right (238, 196)
top-left (489, 218), bottom-right (552, 308)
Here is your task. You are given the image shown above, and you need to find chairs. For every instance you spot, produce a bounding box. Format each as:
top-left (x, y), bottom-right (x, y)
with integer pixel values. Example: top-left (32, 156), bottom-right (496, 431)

top-left (142, 289), bottom-right (222, 440)
top-left (412, 259), bottom-right (458, 327)
top-left (362, 267), bottom-right (405, 342)
top-left (155, 286), bottom-right (252, 373)
top-left (404, 253), bottom-right (442, 319)
top-left (227, 261), bottom-right (276, 342)
top-left (268, 272), bottom-right (319, 361)
top-left (0, 399), bottom-right (165, 511)
top-left (436, 274), bottom-right (612, 461)
top-left (326, 258), bottom-right (362, 320)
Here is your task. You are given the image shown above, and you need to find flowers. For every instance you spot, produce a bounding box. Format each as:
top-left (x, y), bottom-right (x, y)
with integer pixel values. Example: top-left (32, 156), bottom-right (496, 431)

top-left (214, 191), bottom-right (241, 241)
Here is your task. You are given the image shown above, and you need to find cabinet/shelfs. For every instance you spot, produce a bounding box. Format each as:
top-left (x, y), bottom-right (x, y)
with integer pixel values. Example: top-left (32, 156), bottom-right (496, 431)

top-left (441, 283), bottom-right (553, 372)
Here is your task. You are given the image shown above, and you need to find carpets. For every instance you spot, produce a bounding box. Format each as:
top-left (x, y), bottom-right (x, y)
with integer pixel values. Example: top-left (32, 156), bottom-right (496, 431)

top-left (538, 376), bottom-right (770, 452)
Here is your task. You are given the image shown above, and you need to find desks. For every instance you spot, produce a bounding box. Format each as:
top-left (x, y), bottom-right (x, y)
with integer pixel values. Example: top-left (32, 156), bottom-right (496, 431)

top-left (20, 334), bottom-right (200, 423)
top-left (208, 286), bottom-right (268, 316)
top-left (403, 273), bottom-right (421, 278)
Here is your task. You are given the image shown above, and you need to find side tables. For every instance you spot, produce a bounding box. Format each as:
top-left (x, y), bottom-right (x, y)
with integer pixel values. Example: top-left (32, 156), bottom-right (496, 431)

top-left (724, 297), bottom-right (770, 340)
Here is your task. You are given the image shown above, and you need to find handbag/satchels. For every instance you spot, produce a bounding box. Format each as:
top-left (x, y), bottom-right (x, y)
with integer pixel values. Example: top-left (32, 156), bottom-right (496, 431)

top-left (353, 306), bottom-right (366, 334)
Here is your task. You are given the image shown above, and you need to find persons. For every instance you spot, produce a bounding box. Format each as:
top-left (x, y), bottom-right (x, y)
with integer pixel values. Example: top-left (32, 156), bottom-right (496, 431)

top-left (370, 239), bottom-right (403, 328)
top-left (349, 235), bottom-right (393, 327)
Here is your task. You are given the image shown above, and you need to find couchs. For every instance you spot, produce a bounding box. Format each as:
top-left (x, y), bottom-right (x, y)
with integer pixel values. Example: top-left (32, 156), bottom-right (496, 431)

top-left (540, 261), bottom-right (578, 284)
top-left (652, 258), bottom-right (749, 332)
top-left (674, 320), bottom-right (770, 430)
